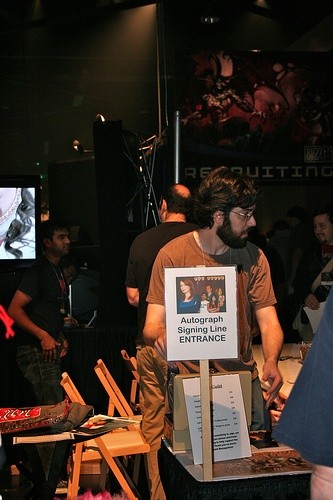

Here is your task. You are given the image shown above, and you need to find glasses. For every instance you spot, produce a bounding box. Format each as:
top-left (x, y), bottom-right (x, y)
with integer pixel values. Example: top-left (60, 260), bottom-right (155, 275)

top-left (230, 209), bottom-right (256, 217)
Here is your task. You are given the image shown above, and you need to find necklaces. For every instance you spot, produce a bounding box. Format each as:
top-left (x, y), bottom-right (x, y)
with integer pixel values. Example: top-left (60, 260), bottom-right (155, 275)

top-left (48, 260), bottom-right (66, 314)
top-left (0, 188), bottom-right (21, 225)
top-left (202, 231), bottom-right (232, 266)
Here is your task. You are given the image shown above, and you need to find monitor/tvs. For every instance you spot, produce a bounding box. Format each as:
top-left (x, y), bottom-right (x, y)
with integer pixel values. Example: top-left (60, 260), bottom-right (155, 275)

top-left (0, 174), bottom-right (41, 274)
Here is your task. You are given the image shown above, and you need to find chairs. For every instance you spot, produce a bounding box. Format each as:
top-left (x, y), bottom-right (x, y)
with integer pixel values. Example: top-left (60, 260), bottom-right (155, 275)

top-left (59, 349), bottom-right (153, 500)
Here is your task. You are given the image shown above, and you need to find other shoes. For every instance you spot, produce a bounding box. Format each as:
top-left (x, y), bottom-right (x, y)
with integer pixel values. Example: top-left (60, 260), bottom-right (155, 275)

top-left (54, 480), bottom-right (80, 494)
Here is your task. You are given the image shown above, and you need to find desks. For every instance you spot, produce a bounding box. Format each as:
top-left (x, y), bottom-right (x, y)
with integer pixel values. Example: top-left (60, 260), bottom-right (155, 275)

top-left (247, 341), bottom-right (318, 403)
top-left (157, 440), bottom-right (313, 500)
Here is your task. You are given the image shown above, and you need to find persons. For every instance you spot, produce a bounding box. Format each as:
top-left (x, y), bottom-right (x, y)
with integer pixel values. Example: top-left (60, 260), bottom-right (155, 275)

top-left (272, 283), bottom-right (333, 500)
top-left (0, 188), bottom-right (35, 259)
top-left (0, 164), bottom-right (333, 500)
top-left (178, 280), bottom-right (226, 314)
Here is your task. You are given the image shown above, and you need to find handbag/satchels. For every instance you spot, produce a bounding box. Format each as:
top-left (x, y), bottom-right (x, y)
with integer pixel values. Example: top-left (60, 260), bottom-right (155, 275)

top-left (293, 305), bottom-right (314, 340)
